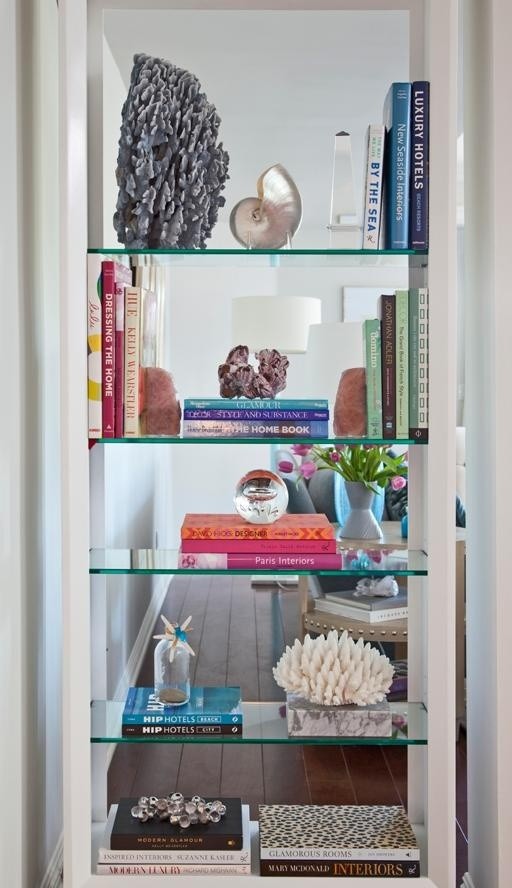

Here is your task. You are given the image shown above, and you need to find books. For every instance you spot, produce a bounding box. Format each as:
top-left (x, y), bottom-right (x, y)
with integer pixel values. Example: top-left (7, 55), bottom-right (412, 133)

top-left (86, 252), bottom-right (157, 440)
top-left (361, 82), bottom-right (429, 251)
top-left (122, 725), bottom-right (243, 735)
top-left (122, 686), bottom-right (242, 725)
top-left (313, 599), bottom-right (407, 624)
top-left (361, 287), bottom-right (428, 439)
top-left (178, 512), bottom-right (342, 570)
top-left (324, 586), bottom-right (407, 611)
top-left (183, 399), bottom-right (329, 438)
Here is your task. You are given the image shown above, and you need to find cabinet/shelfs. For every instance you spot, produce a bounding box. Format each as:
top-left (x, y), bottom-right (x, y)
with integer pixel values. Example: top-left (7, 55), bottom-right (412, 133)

top-left (55, 0), bottom-right (460, 888)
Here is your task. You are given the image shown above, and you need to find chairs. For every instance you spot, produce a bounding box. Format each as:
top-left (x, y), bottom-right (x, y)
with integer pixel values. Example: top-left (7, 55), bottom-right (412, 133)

top-left (298, 575), bottom-right (408, 660)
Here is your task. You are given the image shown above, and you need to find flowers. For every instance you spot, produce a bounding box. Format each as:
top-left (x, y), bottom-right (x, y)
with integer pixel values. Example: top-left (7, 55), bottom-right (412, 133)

top-left (277, 444), bottom-right (408, 498)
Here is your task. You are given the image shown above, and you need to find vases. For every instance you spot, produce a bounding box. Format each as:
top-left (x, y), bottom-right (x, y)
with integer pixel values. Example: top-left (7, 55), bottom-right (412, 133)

top-left (338, 480), bottom-right (386, 542)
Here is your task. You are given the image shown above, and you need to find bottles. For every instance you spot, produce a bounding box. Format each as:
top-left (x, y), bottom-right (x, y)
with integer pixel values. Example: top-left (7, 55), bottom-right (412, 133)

top-left (154, 623), bottom-right (191, 706)
